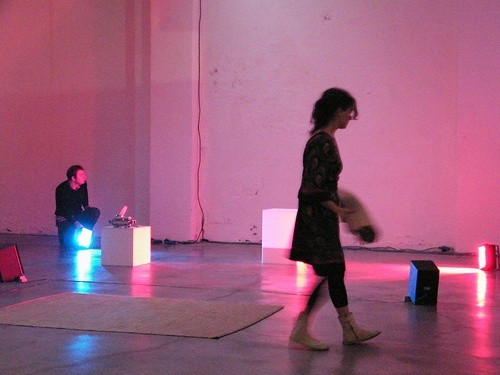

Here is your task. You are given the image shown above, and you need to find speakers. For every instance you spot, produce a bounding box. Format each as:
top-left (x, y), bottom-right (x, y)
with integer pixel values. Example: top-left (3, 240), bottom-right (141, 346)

top-left (408, 259), bottom-right (439, 305)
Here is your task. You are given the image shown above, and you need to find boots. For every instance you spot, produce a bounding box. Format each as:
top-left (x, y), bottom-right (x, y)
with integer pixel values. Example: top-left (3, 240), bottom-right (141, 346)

top-left (289, 310), bottom-right (330, 351)
top-left (337, 311), bottom-right (382, 345)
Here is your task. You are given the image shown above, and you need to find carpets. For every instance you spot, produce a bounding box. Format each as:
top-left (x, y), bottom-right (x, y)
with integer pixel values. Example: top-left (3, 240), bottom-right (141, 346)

top-left (1, 291), bottom-right (285, 339)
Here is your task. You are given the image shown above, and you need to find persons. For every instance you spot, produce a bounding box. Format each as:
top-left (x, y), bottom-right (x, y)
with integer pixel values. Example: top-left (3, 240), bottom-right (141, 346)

top-left (54, 165), bottom-right (100, 250)
top-left (288, 86), bottom-right (383, 352)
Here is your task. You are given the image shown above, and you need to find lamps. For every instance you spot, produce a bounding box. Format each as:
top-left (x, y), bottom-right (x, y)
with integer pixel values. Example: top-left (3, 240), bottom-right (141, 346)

top-left (478, 244), bottom-right (500, 271)
top-left (73, 229), bottom-right (93, 249)
